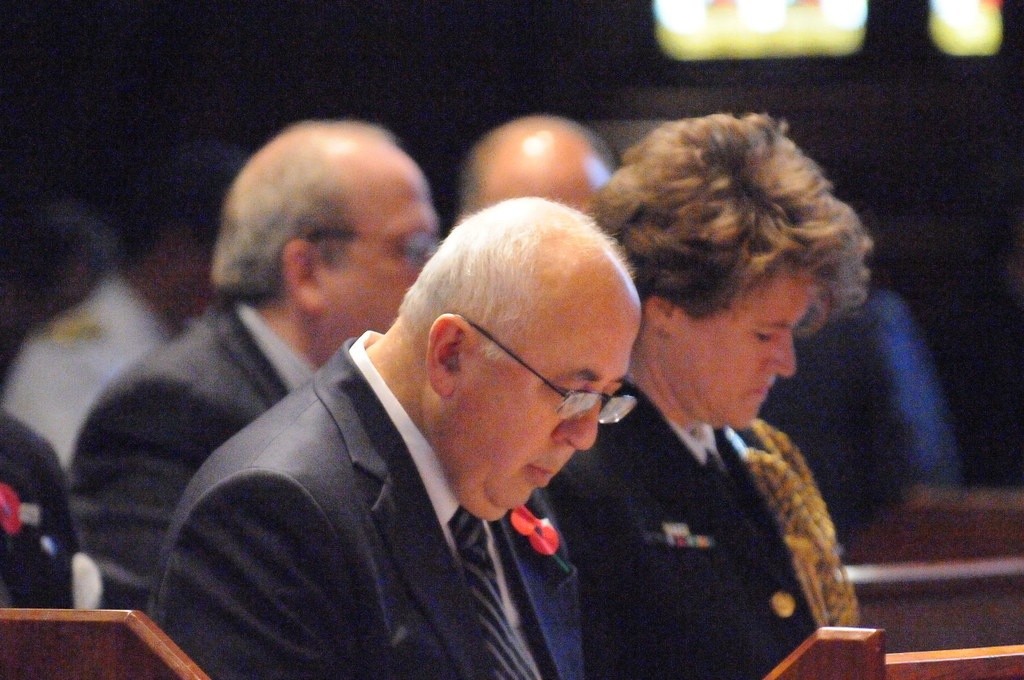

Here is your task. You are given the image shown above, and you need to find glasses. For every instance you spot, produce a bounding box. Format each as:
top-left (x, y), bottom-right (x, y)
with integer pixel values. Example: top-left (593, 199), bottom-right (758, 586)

top-left (468, 323), bottom-right (639, 424)
top-left (306, 221), bottom-right (434, 271)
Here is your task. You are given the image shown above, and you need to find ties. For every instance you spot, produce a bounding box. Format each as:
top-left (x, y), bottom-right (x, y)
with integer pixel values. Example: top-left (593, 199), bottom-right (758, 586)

top-left (448, 504), bottom-right (538, 680)
top-left (704, 450), bottom-right (732, 491)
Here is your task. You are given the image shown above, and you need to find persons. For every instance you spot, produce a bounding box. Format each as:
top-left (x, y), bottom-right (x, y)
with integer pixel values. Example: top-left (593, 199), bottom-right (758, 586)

top-left (3, 137), bottom-right (254, 474)
top-left (453, 114), bottom-right (619, 226)
top-left (65, 118), bottom-right (443, 606)
top-left (540, 113), bottom-right (875, 680)
top-left (145, 196), bottom-right (641, 680)
